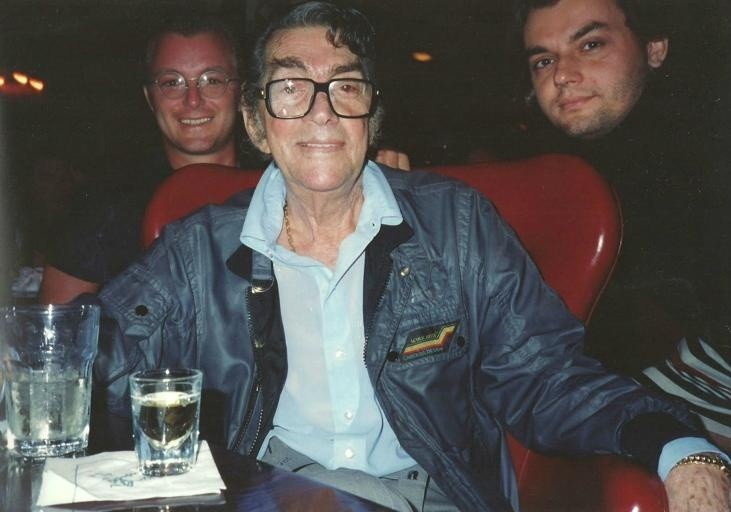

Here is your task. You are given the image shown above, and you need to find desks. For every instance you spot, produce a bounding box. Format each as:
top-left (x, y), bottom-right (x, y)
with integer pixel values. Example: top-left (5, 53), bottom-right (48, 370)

top-left (3, 414), bottom-right (395, 512)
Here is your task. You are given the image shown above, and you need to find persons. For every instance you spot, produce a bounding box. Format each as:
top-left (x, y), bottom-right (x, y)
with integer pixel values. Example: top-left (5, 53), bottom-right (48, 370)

top-left (375, 0), bottom-right (731, 368)
top-left (41, 16), bottom-right (245, 306)
top-left (26, 4), bottom-right (731, 510)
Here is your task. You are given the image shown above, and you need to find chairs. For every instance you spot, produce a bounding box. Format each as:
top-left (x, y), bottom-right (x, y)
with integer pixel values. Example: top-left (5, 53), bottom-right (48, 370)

top-left (137, 148), bottom-right (671, 512)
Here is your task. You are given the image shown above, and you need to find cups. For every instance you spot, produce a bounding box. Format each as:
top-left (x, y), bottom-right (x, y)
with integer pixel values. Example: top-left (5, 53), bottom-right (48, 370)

top-left (0, 302), bottom-right (103, 460)
top-left (131, 367), bottom-right (203, 478)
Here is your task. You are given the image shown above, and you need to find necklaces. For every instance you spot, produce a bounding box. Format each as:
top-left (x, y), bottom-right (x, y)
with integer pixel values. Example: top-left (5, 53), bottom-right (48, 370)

top-left (283, 199), bottom-right (297, 255)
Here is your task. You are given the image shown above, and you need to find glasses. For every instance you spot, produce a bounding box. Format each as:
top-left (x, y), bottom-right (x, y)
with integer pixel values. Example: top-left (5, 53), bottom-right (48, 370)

top-left (150, 71), bottom-right (240, 100)
top-left (260, 78), bottom-right (383, 119)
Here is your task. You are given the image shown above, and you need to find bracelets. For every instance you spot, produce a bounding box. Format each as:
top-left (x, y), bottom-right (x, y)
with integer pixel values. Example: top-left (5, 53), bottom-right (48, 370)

top-left (672, 455), bottom-right (731, 474)
